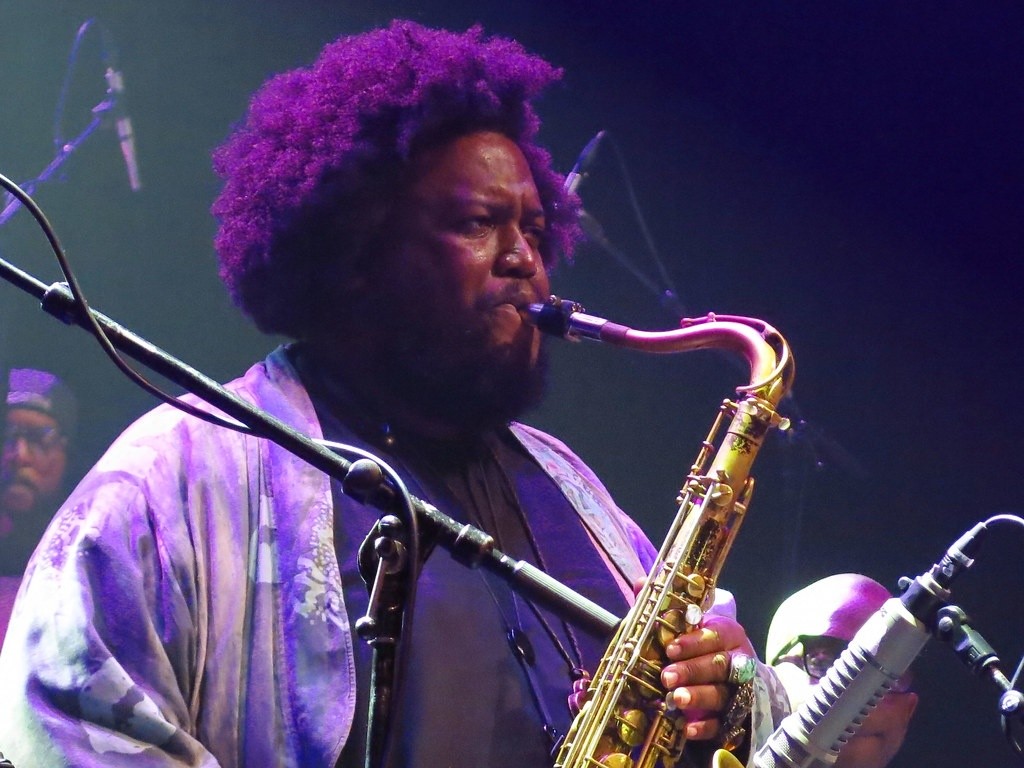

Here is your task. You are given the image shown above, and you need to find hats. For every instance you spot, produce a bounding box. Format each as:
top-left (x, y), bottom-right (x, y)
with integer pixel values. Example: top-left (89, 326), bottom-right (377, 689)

top-left (3, 367), bottom-right (82, 435)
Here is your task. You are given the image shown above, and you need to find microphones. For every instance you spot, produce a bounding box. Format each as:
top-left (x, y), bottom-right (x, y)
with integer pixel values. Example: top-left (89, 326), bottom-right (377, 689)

top-left (744, 518), bottom-right (987, 768)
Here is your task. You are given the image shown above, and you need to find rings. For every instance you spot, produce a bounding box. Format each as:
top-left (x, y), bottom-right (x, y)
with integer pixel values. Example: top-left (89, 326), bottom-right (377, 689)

top-left (728, 652), bottom-right (755, 684)
top-left (722, 684), bottom-right (753, 752)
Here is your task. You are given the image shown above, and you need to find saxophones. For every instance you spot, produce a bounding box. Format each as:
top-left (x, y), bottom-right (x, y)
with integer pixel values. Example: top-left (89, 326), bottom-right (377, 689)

top-left (531, 296), bottom-right (797, 768)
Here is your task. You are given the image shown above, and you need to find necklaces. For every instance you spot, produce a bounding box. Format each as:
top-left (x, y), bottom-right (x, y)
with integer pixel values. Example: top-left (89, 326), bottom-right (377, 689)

top-left (374, 426), bottom-right (597, 722)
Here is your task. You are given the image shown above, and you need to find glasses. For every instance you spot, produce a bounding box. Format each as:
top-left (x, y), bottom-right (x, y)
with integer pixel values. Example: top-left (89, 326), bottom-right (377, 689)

top-left (772, 634), bottom-right (921, 694)
top-left (0, 421), bottom-right (61, 449)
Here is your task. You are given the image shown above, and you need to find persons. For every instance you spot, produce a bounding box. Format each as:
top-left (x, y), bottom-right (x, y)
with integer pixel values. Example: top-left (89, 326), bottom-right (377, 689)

top-left (1, 23), bottom-right (922, 767)
